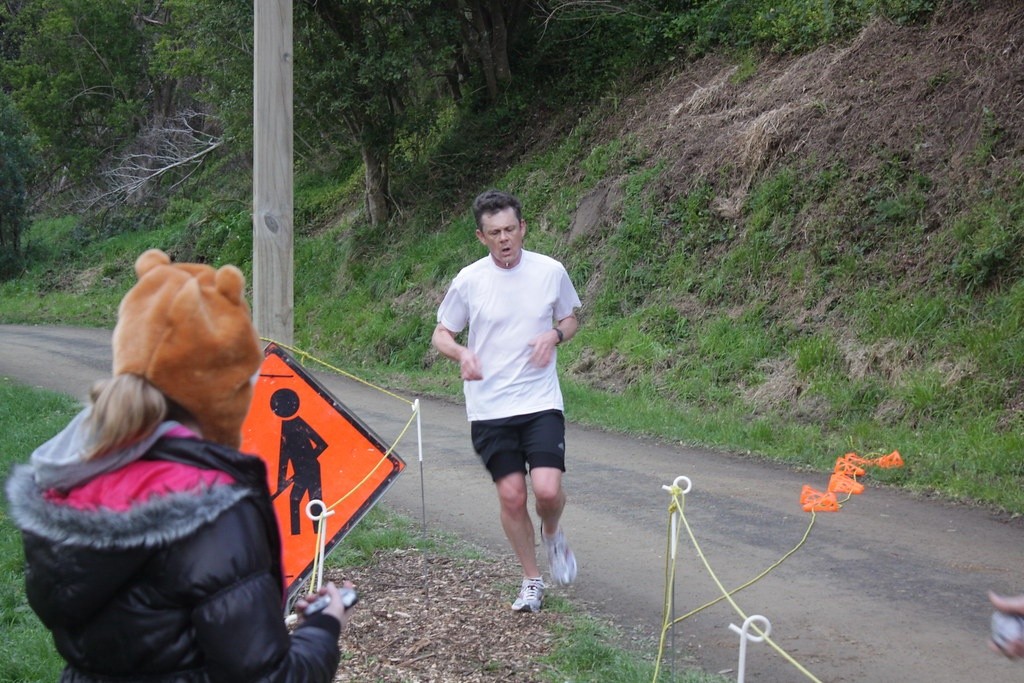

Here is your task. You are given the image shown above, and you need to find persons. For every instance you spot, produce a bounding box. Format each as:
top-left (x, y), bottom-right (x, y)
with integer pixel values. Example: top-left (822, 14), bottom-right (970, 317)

top-left (984, 591), bottom-right (1024, 659)
top-left (5, 249), bottom-right (359, 683)
top-left (431, 189), bottom-right (583, 614)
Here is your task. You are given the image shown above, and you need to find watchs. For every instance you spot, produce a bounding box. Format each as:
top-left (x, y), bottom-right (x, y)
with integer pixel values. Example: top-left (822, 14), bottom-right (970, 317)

top-left (551, 327), bottom-right (563, 346)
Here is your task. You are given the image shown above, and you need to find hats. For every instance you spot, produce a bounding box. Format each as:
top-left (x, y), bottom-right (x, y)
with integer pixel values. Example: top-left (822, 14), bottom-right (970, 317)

top-left (111, 249), bottom-right (262, 449)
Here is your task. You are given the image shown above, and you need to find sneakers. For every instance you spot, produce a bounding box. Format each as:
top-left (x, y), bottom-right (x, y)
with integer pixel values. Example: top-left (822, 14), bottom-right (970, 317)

top-left (511, 575), bottom-right (545, 614)
top-left (539, 521), bottom-right (578, 588)
